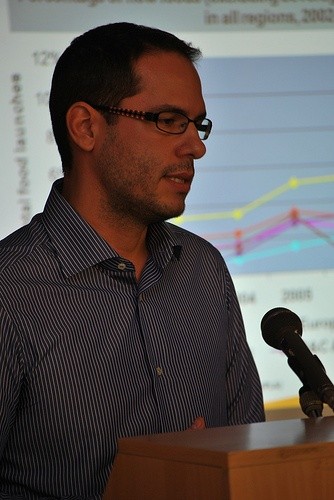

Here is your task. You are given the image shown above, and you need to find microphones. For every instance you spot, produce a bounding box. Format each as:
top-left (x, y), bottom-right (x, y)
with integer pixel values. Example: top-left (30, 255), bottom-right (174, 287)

top-left (260, 307), bottom-right (334, 413)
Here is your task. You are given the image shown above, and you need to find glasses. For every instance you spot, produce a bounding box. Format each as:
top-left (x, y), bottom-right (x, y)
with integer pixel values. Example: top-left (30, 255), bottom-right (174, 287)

top-left (94, 105), bottom-right (212, 140)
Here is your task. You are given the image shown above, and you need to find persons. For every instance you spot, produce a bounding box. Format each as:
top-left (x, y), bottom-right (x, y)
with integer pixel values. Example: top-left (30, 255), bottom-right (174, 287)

top-left (1, 22), bottom-right (265, 496)
